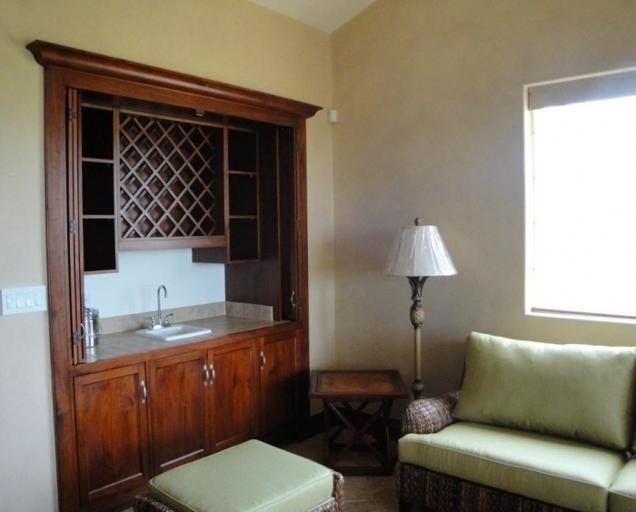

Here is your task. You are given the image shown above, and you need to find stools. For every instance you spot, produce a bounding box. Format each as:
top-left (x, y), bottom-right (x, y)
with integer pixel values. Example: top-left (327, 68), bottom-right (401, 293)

top-left (133, 438), bottom-right (345, 511)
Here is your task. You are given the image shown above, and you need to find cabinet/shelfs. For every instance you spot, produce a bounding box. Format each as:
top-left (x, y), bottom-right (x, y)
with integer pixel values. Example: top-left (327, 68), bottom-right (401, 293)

top-left (257, 321), bottom-right (309, 446)
top-left (81, 102), bottom-right (117, 273)
top-left (145, 330), bottom-right (260, 482)
top-left (52, 353), bottom-right (152, 511)
top-left (228, 126), bottom-right (260, 262)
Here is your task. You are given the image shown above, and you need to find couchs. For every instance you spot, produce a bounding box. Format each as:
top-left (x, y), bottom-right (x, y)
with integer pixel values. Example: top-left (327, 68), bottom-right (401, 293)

top-left (397, 329), bottom-right (635, 511)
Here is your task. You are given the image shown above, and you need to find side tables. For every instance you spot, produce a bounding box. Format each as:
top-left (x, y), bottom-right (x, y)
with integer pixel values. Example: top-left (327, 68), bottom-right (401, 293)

top-left (309, 368), bottom-right (409, 477)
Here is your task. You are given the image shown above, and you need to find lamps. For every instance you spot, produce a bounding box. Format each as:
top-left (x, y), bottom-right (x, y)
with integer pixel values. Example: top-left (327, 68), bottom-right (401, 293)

top-left (388, 217), bottom-right (457, 400)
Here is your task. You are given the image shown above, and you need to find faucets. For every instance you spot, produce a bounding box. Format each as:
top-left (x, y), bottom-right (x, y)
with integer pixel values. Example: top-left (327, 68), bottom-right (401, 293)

top-left (151, 284), bottom-right (175, 329)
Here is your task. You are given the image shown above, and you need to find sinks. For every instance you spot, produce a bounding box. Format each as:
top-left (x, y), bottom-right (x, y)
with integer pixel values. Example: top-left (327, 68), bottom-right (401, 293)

top-left (134, 325), bottom-right (211, 341)
